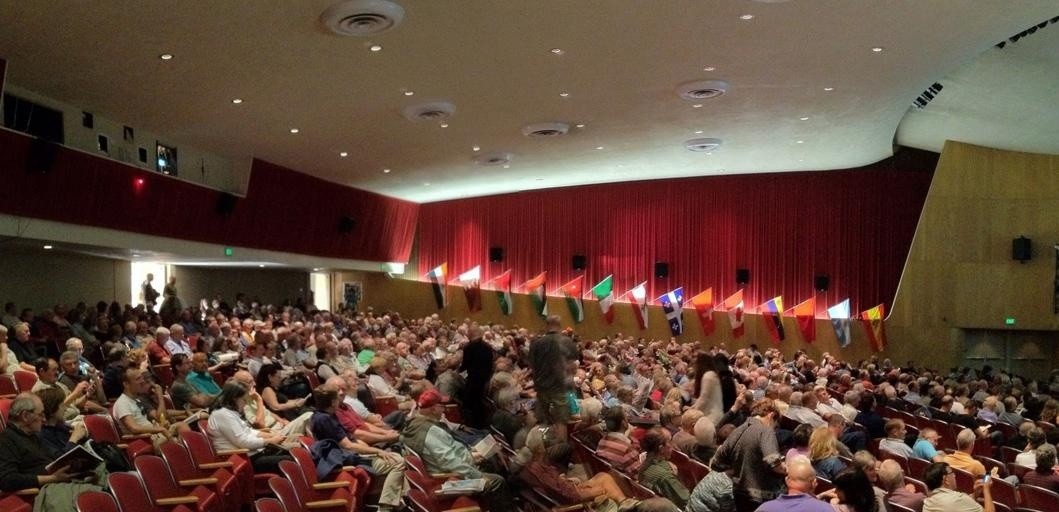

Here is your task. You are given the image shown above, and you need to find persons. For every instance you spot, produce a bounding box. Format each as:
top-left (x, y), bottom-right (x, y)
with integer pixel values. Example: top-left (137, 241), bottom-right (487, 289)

top-left (345, 288), bottom-right (357, 311)
top-left (142, 273), bottom-right (178, 310)
top-left (2, 293), bottom-right (686, 512)
top-left (657, 340), bottom-right (1058, 512)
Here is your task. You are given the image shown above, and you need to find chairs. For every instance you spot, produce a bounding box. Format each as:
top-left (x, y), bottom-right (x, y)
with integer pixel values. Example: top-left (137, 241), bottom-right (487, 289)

top-left (0, 305), bottom-right (1059, 511)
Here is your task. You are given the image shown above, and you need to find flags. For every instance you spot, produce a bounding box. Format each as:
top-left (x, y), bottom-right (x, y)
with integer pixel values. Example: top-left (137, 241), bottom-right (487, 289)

top-left (427, 262), bottom-right (548, 317)
top-left (758, 295), bottom-right (888, 353)
top-left (559, 274), bottom-right (649, 329)
top-left (659, 287), bottom-right (746, 339)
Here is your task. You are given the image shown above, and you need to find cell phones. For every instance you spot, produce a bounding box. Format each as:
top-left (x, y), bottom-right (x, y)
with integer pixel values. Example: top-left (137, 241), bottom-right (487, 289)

top-left (983, 471), bottom-right (990, 482)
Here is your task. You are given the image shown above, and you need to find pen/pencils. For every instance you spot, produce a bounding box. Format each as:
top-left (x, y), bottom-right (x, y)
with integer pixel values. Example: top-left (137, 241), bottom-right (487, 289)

top-left (285, 432), bottom-right (288, 436)
top-left (68, 430), bottom-right (73, 433)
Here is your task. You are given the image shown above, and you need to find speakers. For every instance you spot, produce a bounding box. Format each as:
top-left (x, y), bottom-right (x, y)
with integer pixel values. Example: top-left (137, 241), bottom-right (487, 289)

top-left (491, 247), bottom-right (502, 262)
top-left (656, 261), bottom-right (668, 278)
top-left (736, 270), bottom-right (748, 283)
top-left (1013, 238), bottom-right (1029, 260)
top-left (572, 255), bottom-right (586, 272)
top-left (816, 276), bottom-right (828, 291)
